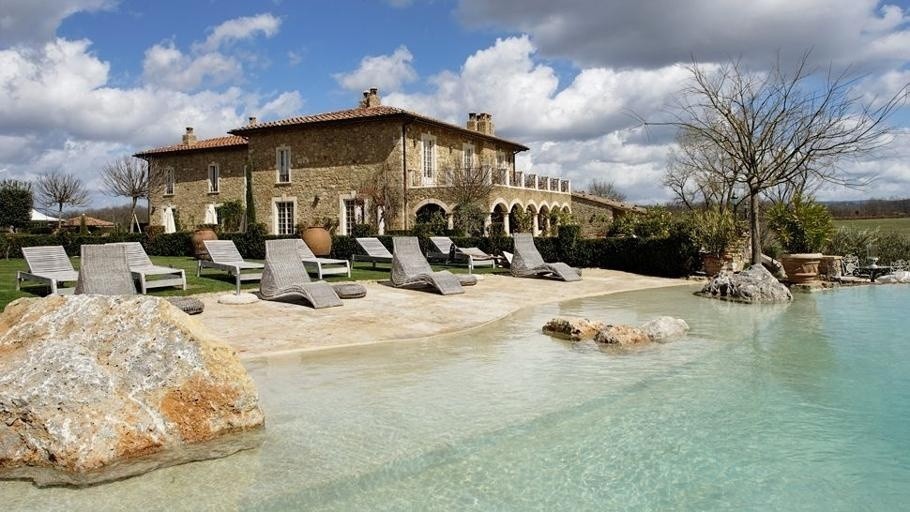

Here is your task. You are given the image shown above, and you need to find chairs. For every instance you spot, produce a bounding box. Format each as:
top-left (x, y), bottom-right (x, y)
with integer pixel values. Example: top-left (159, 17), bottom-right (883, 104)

top-left (16, 245), bottom-right (79, 294)
top-left (74, 244), bottom-right (137, 296)
top-left (260, 239), bottom-right (344, 308)
top-left (197, 239), bottom-right (265, 295)
top-left (390, 236), bottom-right (465, 295)
top-left (425, 232), bottom-right (582, 281)
top-left (104, 242), bottom-right (186, 295)
top-left (282, 239), bottom-right (352, 280)
top-left (350, 237), bottom-right (393, 270)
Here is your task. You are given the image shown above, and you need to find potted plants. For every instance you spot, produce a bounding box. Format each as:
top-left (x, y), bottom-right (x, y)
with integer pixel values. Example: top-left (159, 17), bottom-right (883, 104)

top-left (763, 189), bottom-right (832, 283)
top-left (681, 207), bottom-right (736, 276)
